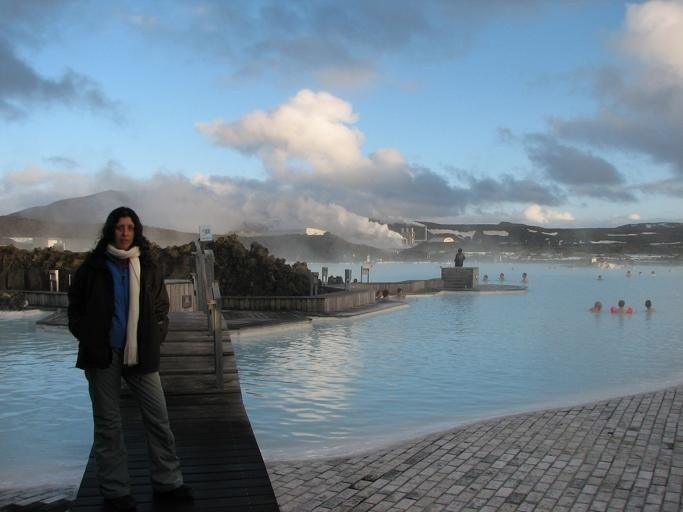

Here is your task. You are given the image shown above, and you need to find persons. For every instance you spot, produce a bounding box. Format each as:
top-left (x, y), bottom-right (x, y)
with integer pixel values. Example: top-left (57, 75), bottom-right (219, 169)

top-left (65, 205), bottom-right (194, 510)
top-left (642, 299), bottom-right (657, 313)
top-left (453, 247), bottom-right (465, 266)
top-left (587, 300), bottom-right (603, 315)
top-left (374, 290), bottom-right (382, 298)
top-left (519, 271), bottom-right (530, 284)
top-left (609, 298), bottom-right (634, 314)
top-left (393, 288), bottom-right (405, 299)
top-left (480, 274), bottom-right (490, 282)
top-left (497, 272), bottom-right (506, 281)
top-left (596, 267), bottom-right (657, 280)
top-left (381, 289), bottom-right (390, 299)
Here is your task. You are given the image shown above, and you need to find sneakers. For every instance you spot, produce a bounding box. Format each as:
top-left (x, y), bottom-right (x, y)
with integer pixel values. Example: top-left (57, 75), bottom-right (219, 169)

top-left (102, 483), bottom-right (192, 512)
